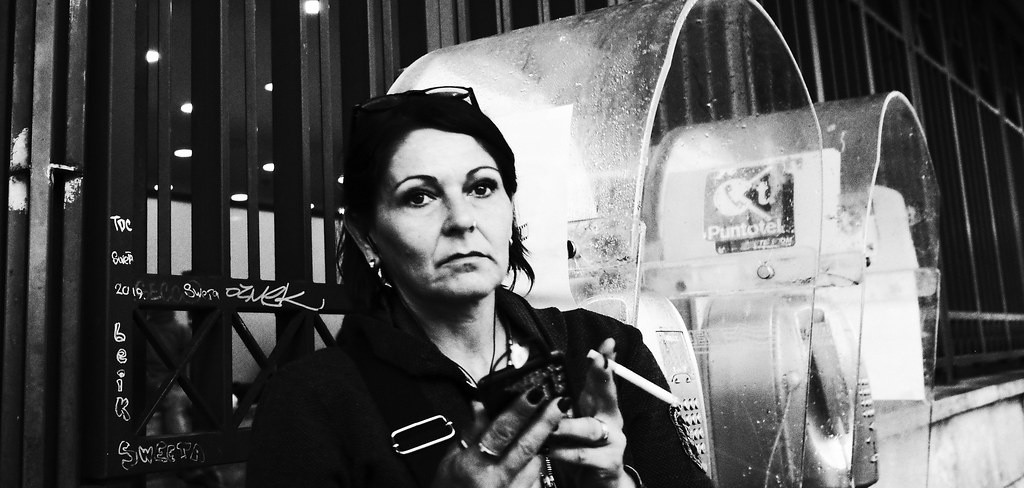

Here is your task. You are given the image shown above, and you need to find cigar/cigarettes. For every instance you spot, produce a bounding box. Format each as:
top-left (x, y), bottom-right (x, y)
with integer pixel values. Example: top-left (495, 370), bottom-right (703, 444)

top-left (582, 342), bottom-right (678, 406)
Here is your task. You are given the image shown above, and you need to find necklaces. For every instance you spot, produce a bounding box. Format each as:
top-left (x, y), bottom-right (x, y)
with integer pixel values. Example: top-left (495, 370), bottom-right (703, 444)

top-left (506, 321), bottom-right (515, 369)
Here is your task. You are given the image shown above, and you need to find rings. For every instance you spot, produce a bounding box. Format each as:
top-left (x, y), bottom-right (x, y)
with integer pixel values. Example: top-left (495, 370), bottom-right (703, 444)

top-left (592, 415), bottom-right (611, 443)
top-left (468, 433), bottom-right (510, 465)
top-left (453, 431), bottom-right (467, 452)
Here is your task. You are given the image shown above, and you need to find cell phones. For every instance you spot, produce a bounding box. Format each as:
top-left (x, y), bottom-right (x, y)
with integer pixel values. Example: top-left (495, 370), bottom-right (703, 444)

top-left (474, 350), bottom-right (583, 421)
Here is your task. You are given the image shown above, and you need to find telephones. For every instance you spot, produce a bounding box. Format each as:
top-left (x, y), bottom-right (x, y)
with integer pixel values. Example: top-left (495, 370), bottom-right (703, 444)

top-left (571, 291), bottom-right (712, 478)
top-left (702, 295), bottom-right (879, 488)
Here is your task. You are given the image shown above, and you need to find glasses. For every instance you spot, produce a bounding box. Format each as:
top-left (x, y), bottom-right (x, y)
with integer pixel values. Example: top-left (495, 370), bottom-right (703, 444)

top-left (346, 86), bottom-right (479, 144)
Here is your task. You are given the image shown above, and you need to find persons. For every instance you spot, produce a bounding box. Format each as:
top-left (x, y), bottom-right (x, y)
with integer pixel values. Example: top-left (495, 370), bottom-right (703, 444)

top-left (251, 79), bottom-right (720, 488)
